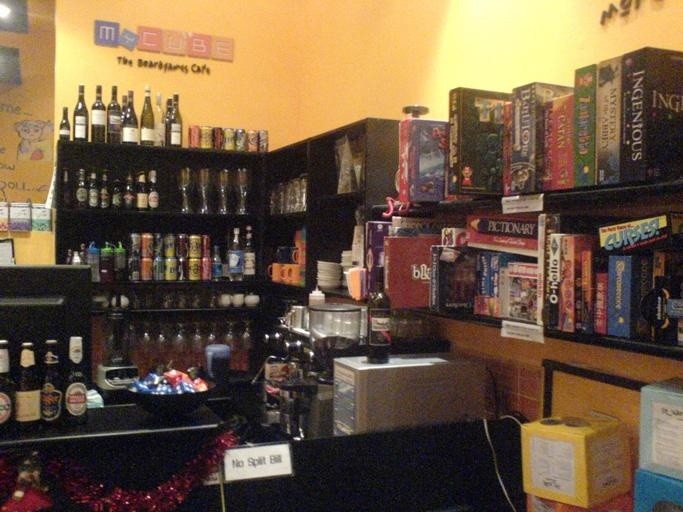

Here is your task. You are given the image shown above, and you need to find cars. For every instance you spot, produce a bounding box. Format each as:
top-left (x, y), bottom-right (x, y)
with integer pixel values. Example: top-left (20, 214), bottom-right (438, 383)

top-left (268, 225), bottom-right (306, 288)
top-left (343, 267), bottom-right (365, 301)
top-left (352, 225), bottom-right (364, 267)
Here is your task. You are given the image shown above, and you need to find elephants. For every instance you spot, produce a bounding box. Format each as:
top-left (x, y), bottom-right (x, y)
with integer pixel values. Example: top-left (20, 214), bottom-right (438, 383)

top-left (31, 148), bottom-right (44, 160)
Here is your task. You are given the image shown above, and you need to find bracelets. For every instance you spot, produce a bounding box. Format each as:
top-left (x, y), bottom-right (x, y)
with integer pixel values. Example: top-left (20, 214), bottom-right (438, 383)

top-left (126, 379), bottom-right (217, 412)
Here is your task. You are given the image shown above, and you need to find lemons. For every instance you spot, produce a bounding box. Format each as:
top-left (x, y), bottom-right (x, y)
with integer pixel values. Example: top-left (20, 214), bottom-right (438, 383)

top-left (218, 292), bottom-right (260, 308)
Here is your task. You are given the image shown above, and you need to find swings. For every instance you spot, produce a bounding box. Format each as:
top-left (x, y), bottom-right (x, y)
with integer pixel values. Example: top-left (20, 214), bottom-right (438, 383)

top-left (300, 304), bottom-right (361, 440)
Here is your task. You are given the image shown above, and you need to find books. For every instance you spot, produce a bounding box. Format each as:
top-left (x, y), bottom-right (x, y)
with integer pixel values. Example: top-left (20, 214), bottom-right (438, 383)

top-left (520, 413), bottom-right (632, 508)
top-left (640, 379), bottom-right (683, 479)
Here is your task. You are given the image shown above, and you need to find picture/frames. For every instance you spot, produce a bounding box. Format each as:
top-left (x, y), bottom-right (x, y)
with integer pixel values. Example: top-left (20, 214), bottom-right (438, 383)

top-left (0, 402), bottom-right (224, 450)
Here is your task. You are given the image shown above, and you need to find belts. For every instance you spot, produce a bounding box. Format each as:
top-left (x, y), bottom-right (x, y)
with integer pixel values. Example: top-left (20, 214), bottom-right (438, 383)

top-left (175, 165), bottom-right (252, 217)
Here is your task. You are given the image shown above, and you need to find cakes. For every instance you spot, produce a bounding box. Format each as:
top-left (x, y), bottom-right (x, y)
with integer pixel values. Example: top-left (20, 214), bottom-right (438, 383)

top-left (127, 233), bottom-right (212, 281)
top-left (189, 125), bottom-right (268, 152)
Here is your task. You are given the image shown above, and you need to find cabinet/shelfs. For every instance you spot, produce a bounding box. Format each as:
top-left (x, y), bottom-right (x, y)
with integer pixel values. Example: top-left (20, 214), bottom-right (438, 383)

top-left (60, 84), bottom-right (183, 209)
top-left (214, 224), bottom-right (256, 283)
top-left (66, 240), bottom-right (126, 283)
top-left (266, 172), bottom-right (309, 214)
top-left (111, 295), bottom-right (130, 310)
top-left (102, 321), bottom-right (256, 377)
top-left (264, 351), bottom-right (318, 438)
top-left (0, 202), bottom-right (52, 234)
top-left (365, 265), bottom-right (391, 363)
top-left (284, 287), bottom-right (325, 331)
top-left (0, 336), bottom-right (88, 432)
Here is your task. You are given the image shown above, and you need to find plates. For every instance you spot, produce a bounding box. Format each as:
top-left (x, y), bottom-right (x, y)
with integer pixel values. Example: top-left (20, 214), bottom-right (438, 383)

top-left (371, 174), bottom-right (683, 363)
top-left (56, 141), bottom-right (259, 314)
top-left (260, 116), bottom-right (397, 356)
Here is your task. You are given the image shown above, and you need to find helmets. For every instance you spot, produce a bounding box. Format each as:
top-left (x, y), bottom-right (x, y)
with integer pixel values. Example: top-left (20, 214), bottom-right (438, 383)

top-left (316, 251), bottom-right (352, 290)
